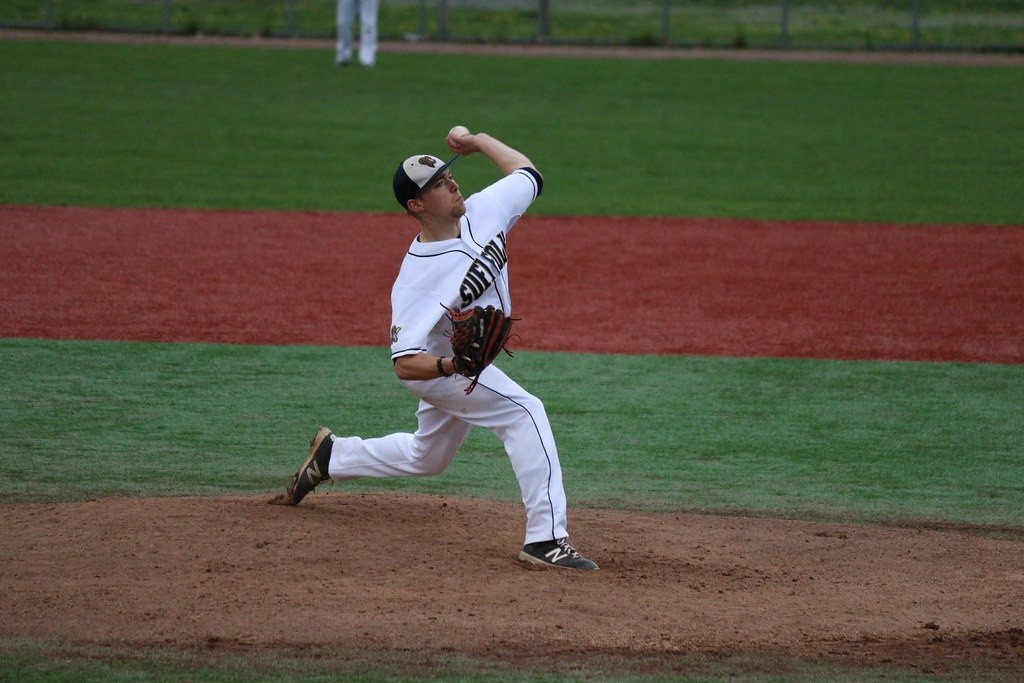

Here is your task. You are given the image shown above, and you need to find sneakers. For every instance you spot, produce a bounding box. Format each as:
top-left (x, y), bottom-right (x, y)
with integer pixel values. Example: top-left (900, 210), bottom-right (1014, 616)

top-left (519, 537), bottom-right (600, 571)
top-left (288, 427), bottom-right (338, 506)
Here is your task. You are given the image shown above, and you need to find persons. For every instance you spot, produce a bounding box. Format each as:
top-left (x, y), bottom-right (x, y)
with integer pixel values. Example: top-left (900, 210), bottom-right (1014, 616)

top-left (335, 0), bottom-right (378, 65)
top-left (288, 132), bottom-right (599, 571)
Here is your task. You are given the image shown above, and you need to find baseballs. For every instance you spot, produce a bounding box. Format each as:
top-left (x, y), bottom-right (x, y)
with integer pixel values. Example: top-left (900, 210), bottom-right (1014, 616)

top-left (448, 125), bottom-right (470, 148)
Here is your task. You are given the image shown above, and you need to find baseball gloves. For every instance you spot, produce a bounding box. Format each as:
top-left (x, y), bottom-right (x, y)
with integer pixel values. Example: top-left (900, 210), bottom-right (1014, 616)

top-left (438, 301), bottom-right (524, 397)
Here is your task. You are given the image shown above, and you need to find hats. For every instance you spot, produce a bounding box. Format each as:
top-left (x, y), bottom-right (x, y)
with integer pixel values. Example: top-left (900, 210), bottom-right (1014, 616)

top-left (393, 155), bottom-right (460, 206)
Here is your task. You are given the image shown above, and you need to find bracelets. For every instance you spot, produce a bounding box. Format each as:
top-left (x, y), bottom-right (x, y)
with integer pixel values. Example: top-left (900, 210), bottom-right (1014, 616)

top-left (437, 356), bottom-right (453, 377)
